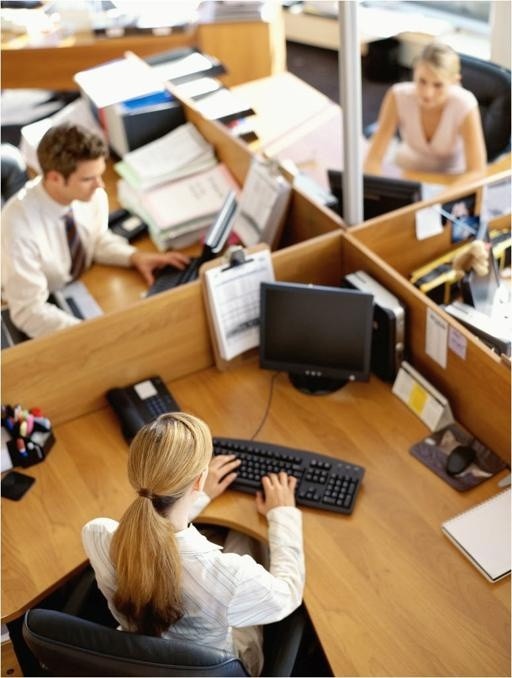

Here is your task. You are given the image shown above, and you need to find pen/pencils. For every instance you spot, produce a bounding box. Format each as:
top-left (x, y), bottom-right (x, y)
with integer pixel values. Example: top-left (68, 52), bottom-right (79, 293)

top-left (20, 421), bottom-right (28, 438)
top-left (15, 439), bottom-right (28, 455)
top-left (7, 403), bottom-right (28, 429)
top-left (26, 414), bottom-right (36, 435)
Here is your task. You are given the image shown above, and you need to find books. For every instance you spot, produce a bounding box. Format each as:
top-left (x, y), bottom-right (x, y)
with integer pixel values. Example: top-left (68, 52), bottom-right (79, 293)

top-left (117, 125), bottom-right (234, 251)
top-left (207, 251), bottom-right (277, 361)
top-left (445, 298), bottom-right (510, 355)
top-left (442, 487), bottom-right (512, 583)
top-left (232, 153), bottom-right (291, 250)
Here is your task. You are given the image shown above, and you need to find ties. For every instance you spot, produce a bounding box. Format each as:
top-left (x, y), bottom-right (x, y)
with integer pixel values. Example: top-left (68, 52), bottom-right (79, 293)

top-left (64, 212), bottom-right (87, 282)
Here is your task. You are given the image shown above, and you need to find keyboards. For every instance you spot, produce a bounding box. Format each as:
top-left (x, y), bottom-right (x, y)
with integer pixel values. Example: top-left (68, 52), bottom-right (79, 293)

top-left (211, 436), bottom-right (365, 516)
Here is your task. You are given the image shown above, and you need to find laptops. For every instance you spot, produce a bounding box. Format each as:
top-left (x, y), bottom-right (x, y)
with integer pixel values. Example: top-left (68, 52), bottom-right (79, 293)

top-left (144, 194), bottom-right (238, 296)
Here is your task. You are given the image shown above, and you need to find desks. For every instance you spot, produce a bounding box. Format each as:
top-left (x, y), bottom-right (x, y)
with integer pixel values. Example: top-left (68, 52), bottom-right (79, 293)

top-left (161, 71), bottom-right (452, 228)
top-left (0, 230), bottom-right (511, 678)
top-left (1, 20), bottom-right (273, 89)
top-left (342, 170), bottom-right (512, 362)
top-left (26, 50), bottom-right (344, 315)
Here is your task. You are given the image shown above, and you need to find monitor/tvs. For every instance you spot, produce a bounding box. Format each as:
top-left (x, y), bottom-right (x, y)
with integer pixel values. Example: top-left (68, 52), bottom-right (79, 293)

top-left (259, 282), bottom-right (373, 397)
top-left (327, 168), bottom-right (421, 221)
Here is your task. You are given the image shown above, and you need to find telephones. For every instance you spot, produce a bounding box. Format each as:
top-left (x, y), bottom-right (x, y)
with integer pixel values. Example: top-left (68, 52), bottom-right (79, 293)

top-left (105, 375), bottom-right (182, 447)
top-left (108, 208), bottom-right (146, 240)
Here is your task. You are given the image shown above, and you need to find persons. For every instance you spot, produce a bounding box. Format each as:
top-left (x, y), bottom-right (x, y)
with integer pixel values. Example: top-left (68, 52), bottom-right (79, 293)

top-left (361, 43), bottom-right (488, 193)
top-left (1, 124), bottom-right (190, 340)
top-left (80, 412), bottom-right (307, 678)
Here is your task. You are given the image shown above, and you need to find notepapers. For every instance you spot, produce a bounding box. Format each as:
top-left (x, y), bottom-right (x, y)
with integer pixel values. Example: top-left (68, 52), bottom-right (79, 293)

top-left (447, 327), bottom-right (467, 361)
top-left (414, 204), bottom-right (444, 241)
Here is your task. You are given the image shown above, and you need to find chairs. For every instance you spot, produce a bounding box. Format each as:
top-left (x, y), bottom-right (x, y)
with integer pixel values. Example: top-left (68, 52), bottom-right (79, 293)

top-left (2, 141), bottom-right (30, 201)
top-left (460, 50), bottom-right (510, 162)
top-left (21, 570), bottom-right (307, 676)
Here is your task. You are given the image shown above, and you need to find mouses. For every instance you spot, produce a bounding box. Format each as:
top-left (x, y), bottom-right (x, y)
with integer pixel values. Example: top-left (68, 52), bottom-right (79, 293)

top-left (445, 444), bottom-right (477, 476)
